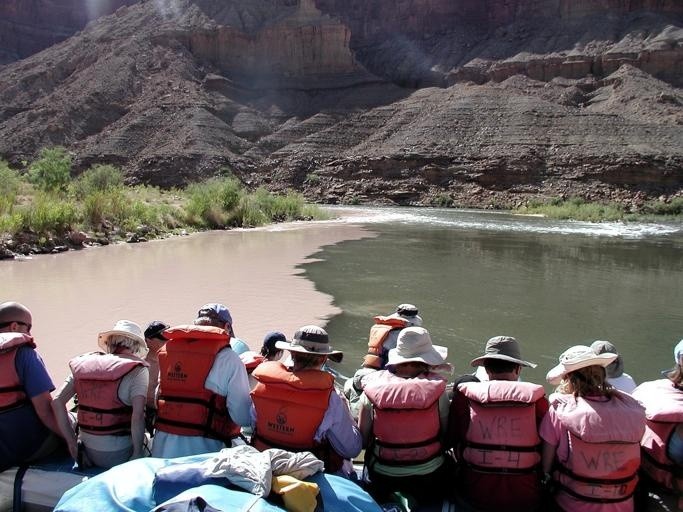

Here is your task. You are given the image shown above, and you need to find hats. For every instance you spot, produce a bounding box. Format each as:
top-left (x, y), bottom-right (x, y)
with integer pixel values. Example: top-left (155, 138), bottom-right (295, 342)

top-left (546, 345), bottom-right (617, 385)
top-left (98, 320), bottom-right (148, 358)
top-left (674, 340), bottom-right (683, 365)
top-left (264, 332), bottom-right (286, 347)
top-left (385, 327), bottom-right (448, 366)
top-left (590, 340), bottom-right (624, 378)
top-left (198, 303), bottom-right (235, 339)
top-left (144, 322), bottom-right (170, 342)
top-left (275, 326), bottom-right (343, 363)
top-left (388, 304), bottom-right (424, 326)
top-left (471, 336), bottom-right (539, 368)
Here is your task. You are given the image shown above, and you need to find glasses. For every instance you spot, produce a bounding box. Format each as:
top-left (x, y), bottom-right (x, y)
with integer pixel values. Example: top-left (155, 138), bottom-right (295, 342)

top-left (0, 321), bottom-right (32, 333)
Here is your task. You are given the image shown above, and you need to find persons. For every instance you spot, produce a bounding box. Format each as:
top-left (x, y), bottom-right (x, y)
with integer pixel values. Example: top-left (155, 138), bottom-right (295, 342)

top-left (361, 303), bottom-right (423, 369)
top-left (51, 318), bottom-right (150, 471)
top-left (238, 330), bottom-right (286, 390)
top-left (590, 340), bottom-right (637, 392)
top-left (250, 324), bottom-right (362, 474)
top-left (631, 336), bottom-right (683, 512)
top-left (151, 303), bottom-right (249, 458)
top-left (145, 321), bottom-right (169, 412)
top-left (539, 344), bottom-right (645, 512)
top-left (358, 326), bottom-right (450, 512)
top-left (0, 301), bottom-right (77, 471)
top-left (451, 334), bottom-right (549, 512)
